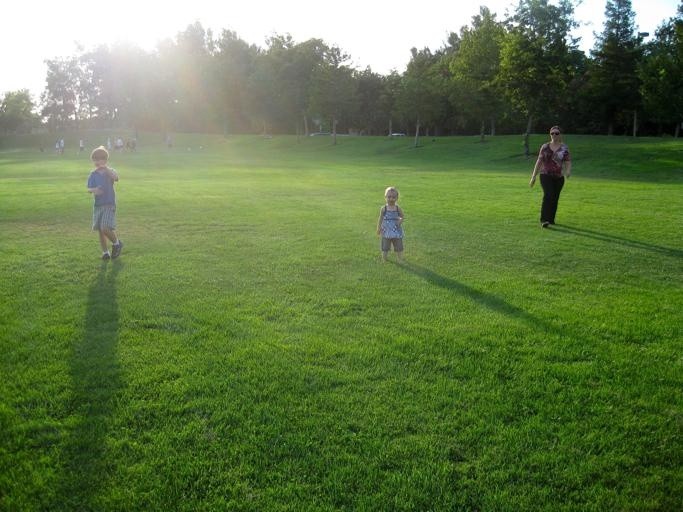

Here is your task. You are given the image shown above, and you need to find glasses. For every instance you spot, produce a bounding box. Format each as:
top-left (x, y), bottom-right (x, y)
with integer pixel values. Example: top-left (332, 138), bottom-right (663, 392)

top-left (550, 132), bottom-right (559, 135)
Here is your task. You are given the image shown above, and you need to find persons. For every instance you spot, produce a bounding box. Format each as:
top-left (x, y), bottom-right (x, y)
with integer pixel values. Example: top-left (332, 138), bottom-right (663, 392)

top-left (166, 135), bottom-right (175, 153)
top-left (55, 138), bottom-right (60, 155)
top-left (529, 125), bottom-right (571, 228)
top-left (86, 146), bottom-right (124, 260)
top-left (78, 138), bottom-right (85, 156)
top-left (376, 187), bottom-right (405, 264)
top-left (106, 136), bottom-right (136, 153)
top-left (59, 138), bottom-right (65, 154)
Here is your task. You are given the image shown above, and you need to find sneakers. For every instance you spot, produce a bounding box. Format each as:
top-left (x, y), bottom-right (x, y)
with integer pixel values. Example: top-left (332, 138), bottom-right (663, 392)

top-left (541, 221), bottom-right (549, 228)
top-left (103, 241), bottom-right (123, 259)
